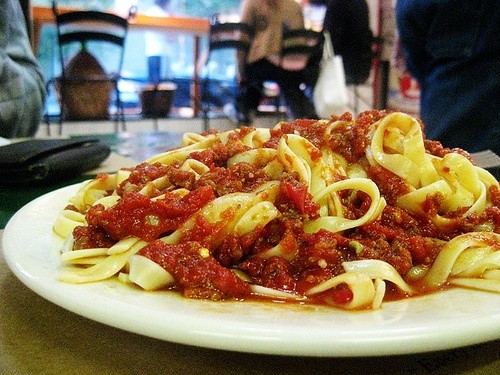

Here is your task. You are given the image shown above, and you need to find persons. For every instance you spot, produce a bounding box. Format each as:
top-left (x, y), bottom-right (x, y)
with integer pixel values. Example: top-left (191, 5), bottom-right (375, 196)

top-left (0, 0), bottom-right (50, 141)
top-left (233, 0), bottom-right (500, 184)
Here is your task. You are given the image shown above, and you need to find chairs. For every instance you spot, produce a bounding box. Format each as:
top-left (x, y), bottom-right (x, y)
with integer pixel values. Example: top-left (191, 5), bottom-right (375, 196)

top-left (278, 22), bottom-right (326, 118)
top-left (204, 14), bottom-right (257, 132)
top-left (44, 0), bottom-right (128, 134)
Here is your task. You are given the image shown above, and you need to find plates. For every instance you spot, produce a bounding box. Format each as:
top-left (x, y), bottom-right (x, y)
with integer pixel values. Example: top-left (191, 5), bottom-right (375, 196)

top-left (2, 179), bottom-right (500, 358)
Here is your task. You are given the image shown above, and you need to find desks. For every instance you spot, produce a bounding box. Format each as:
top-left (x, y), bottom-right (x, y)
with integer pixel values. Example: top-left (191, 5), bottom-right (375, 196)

top-left (33, 5), bottom-right (212, 119)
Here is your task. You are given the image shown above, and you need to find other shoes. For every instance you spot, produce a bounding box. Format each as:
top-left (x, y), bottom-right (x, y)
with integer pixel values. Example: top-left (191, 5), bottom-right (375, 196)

top-left (237, 109), bottom-right (255, 127)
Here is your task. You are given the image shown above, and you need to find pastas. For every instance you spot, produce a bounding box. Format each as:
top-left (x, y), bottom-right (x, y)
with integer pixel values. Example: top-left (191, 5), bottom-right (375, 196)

top-left (53, 109), bottom-right (500, 310)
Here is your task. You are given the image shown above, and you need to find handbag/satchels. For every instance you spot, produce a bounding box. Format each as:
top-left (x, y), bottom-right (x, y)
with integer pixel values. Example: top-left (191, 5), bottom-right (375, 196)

top-left (0, 139), bottom-right (111, 183)
